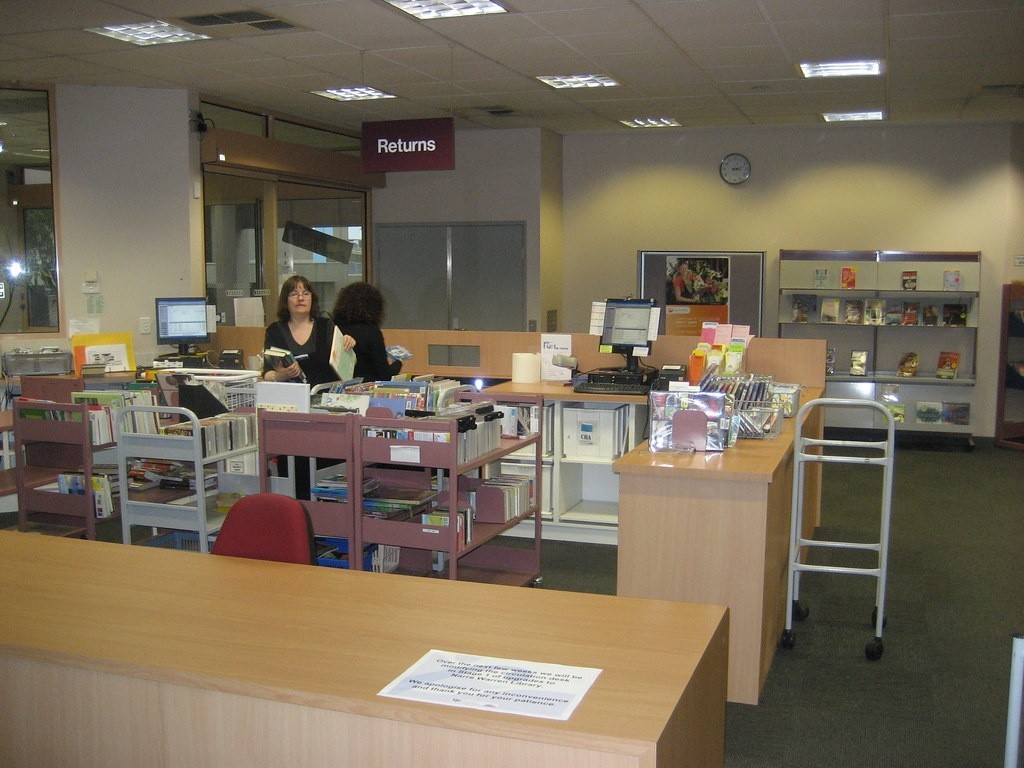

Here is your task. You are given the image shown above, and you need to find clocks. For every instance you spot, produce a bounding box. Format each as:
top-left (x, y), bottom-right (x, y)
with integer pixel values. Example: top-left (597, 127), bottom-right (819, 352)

top-left (719, 153), bottom-right (752, 186)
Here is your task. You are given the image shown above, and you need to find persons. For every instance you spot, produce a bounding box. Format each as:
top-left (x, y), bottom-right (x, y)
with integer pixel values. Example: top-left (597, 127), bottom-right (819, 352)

top-left (261, 275), bottom-right (356, 393)
top-left (673, 261), bottom-right (708, 304)
top-left (332, 280), bottom-right (403, 385)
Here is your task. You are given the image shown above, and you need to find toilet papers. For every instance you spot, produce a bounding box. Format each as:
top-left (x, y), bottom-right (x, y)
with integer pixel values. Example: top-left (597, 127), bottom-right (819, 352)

top-left (511, 352), bottom-right (541, 384)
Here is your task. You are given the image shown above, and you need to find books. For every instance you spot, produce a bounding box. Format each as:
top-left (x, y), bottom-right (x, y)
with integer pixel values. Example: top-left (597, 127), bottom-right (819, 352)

top-left (0, 326), bottom-right (533, 572)
top-left (648, 374), bottom-right (769, 452)
top-left (791, 267), bottom-right (972, 425)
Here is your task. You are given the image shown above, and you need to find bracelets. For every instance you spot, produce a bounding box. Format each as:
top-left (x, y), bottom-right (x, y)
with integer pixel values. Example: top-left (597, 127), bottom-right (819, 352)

top-left (273, 372), bottom-right (277, 382)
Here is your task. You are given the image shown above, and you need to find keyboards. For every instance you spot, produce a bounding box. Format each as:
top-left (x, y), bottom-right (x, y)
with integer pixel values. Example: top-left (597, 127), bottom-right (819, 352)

top-left (588, 368), bottom-right (659, 386)
top-left (574, 383), bottom-right (650, 395)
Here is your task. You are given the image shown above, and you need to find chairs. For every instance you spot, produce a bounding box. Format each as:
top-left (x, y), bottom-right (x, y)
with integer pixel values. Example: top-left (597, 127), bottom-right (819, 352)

top-left (209, 491), bottom-right (322, 569)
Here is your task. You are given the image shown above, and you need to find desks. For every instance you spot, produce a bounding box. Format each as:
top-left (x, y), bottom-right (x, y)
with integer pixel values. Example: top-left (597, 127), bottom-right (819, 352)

top-left (0, 528), bottom-right (731, 768)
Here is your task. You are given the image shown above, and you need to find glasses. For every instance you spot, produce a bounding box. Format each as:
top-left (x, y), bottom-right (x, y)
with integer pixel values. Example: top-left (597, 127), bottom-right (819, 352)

top-left (287, 289), bottom-right (312, 299)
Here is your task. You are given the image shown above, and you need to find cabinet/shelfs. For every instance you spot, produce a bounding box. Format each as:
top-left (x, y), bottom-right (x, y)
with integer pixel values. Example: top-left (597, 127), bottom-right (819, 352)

top-left (0, 365), bottom-right (828, 706)
top-left (780, 248), bottom-right (982, 445)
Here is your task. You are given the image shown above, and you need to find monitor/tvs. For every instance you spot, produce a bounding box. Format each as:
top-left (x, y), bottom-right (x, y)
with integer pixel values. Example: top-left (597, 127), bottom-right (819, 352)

top-left (155, 297), bottom-right (211, 356)
top-left (597, 298), bottom-right (656, 374)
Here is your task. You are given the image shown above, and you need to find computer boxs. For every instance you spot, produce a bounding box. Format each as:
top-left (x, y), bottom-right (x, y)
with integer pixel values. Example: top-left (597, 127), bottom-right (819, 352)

top-left (153, 353), bottom-right (208, 369)
